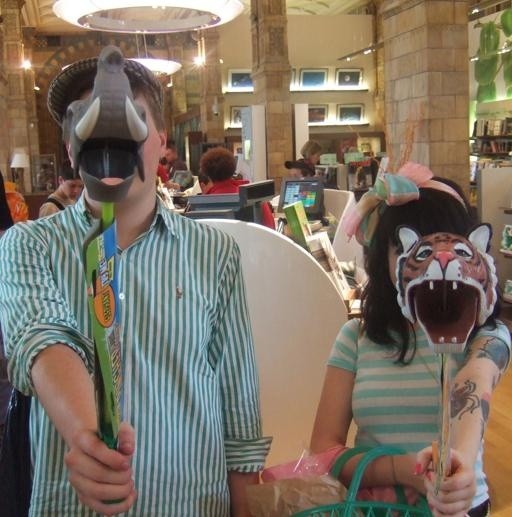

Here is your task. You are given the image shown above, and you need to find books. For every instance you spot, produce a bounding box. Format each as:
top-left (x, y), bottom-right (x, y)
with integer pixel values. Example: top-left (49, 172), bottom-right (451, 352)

top-left (471, 115), bottom-right (512, 153)
top-left (315, 162), bottom-right (349, 192)
top-left (307, 230), bottom-right (352, 300)
top-left (283, 200), bottom-right (313, 250)
top-left (390, 456), bottom-right (399, 486)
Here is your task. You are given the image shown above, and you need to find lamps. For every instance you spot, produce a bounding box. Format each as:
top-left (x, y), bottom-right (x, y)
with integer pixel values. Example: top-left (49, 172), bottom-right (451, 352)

top-left (10, 154), bottom-right (30, 193)
top-left (123, 33), bottom-right (182, 84)
top-left (53, 0), bottom-right (245, 33)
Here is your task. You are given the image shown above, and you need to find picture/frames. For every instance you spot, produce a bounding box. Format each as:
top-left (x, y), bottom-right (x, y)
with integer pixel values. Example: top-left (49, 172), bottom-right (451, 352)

top-left (291, 68), bottom-right (296, 87)
top-left (336, 103), bottom-right (365, 123)
top-left (308, 103), bottom-right (328, 123)
top-left (335, 68), bottom-right (362, 88)
top-left (228, 68), bottom-right (253, 89)
top-left (230, 105), bottom-right (248, 126)
top-left (299, 68), bottom-right (328, 88)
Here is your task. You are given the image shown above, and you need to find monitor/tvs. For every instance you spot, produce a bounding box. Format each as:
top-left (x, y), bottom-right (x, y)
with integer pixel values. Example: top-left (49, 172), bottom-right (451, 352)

top-left (183, 195), bottom-right (250, 227)
top-left (307, 188), bottom-right (310, 190)
top-left (276, 176), bottom-right (324, 214)
top-left (173, 170), bottom-right (192, 187)
top-left (239, 179), bottom-right (274, 203)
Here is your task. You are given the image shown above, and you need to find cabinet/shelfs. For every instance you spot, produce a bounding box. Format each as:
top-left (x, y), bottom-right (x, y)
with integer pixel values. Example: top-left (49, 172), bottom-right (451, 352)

top-left (469, 121), bottom-right (512, 157)
top-left (223, 89), bottom-right (370, 129)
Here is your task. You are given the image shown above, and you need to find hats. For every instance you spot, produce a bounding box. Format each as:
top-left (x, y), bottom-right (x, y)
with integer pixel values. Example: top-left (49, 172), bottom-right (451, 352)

top-left (47, 57), bottom-right (164, 129)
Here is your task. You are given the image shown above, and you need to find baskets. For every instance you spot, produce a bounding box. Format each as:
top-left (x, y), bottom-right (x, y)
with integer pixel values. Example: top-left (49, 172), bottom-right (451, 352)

top-left (288, 442), bottom-right (434, 517)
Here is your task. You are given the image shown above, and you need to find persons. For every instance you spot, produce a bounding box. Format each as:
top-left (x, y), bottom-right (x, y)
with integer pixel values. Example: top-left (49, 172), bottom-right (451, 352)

top-left (200, 147), bottom-right (276, 231)
top-left (39, 161), bottom-right (83, 218)
top-left (309, 163), bottom-right (511, 517)
top-left (0, 54), bottom-right (273, 517)
top-left (165, 145), bottom-right (187, 180)
top-left (158, 156), bottom-right (169, 185)
top-left (197, 172), bottom-right (214, 195)
top-left (300, 140), bottom-right (322, 167)
